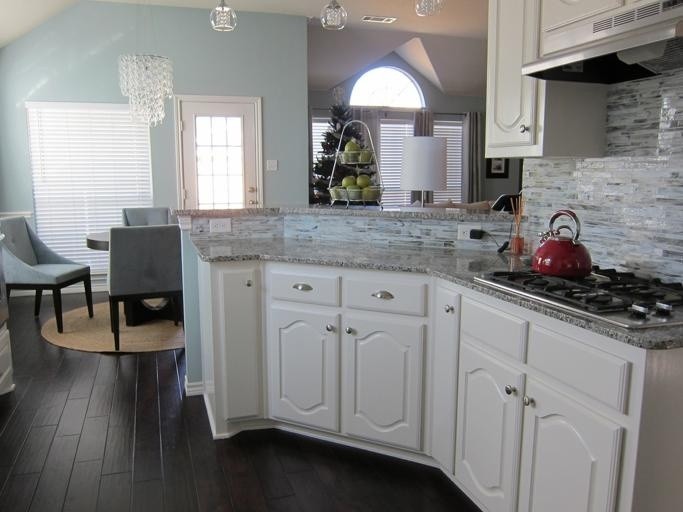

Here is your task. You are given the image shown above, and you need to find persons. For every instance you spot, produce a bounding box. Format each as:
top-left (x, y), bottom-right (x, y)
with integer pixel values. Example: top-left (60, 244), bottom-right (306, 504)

top-left (404, 198), bottom-right (490, 210)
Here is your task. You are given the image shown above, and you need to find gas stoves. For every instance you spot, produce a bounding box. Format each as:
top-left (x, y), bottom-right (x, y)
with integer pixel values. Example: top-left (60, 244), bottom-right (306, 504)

top-left (473, 266), bottom-right (683, 331)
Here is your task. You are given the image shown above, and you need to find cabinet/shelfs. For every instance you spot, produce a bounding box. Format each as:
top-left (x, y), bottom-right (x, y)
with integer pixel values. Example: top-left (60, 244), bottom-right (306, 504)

top-left (431, 271), bottom-right (683, 512)
top-left (197, 249), bottom-right (429, 466)
top-left (485, 0), bottom-right (606, 158)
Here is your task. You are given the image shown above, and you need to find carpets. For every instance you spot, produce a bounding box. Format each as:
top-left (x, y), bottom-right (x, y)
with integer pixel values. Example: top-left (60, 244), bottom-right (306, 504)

top-left (41, 298), bottom-right (184, 352)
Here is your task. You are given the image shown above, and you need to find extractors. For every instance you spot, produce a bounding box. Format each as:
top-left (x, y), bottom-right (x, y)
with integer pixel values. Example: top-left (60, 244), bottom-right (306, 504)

top-left (521, 2), bottom-right (683, 88)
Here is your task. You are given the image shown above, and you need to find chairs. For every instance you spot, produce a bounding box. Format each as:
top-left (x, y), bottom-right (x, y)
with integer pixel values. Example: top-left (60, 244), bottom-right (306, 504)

top-left (0, 217), bottom-right (94, 333)
top-left (122, 207), bottom-right (174, 226)
top-left (108, 225), bottom-right (183, 351)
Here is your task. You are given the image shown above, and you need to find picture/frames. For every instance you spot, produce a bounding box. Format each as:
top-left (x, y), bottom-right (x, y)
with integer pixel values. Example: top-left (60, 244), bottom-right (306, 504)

top-left (485, 158), bottom-right (509, 180)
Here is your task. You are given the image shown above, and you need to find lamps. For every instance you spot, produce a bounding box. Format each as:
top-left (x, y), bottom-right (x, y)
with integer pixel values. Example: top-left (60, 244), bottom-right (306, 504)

top-left (400, 136), bottom-right (448, 212)
top-left (414, 0), bottom-right (445, 16)
top-left (118, 54), bottom-right (174, 128)
top-left (320, 0), bottom-right (347, 31)
top-left (209, 0), bottom-right (237, 32)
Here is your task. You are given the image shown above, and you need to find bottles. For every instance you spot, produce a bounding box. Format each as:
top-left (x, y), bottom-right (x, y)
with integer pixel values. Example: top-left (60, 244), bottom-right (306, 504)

top-left (509, 255), bottom-right (524, 271)
top-left (509, 233), bottom-right (525, 254)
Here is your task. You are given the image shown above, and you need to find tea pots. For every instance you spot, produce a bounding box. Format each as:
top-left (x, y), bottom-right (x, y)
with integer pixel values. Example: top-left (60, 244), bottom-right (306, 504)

top-left (530, 209), bottom-right (592, 279)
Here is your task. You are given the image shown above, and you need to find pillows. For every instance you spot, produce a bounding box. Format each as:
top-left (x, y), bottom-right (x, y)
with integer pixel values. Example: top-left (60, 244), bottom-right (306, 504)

top-left (453, 200), bottom-right (490, 212)
top-left (424, 200), bottom-right (454, 208)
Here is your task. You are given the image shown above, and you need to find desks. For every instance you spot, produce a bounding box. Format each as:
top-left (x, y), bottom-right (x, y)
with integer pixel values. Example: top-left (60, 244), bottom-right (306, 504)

top-left (86, 233), bottom-right (183, 325)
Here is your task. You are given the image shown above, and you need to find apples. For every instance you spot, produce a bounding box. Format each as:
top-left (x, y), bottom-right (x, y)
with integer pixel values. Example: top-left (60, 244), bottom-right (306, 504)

top-left (341, 141), bottom-right (371, 162)
top-left (330, 175), bottom-right (380, 201)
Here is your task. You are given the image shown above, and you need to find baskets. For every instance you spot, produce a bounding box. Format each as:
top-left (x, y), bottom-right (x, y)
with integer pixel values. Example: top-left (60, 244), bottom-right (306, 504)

top-left (328, 185), bottom-right (384, 202)
top-left (337, 149), bottom-right (375, 165)
